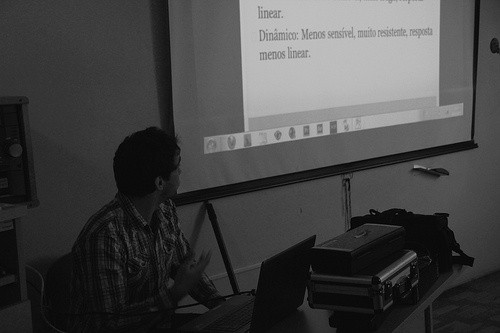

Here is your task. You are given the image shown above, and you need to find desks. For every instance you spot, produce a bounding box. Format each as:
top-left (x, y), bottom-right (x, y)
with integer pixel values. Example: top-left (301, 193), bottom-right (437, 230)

top-left (260, 263), bottom-right (456, 333)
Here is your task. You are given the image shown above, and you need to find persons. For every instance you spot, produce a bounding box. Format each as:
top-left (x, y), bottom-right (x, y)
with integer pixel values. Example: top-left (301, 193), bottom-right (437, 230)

top-left (52, 127), bottom-right (227, 332)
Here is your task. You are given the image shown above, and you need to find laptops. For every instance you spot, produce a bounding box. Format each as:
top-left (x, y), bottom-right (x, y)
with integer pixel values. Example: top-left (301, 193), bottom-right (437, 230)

top-left (174, 234), bottom-right (316, 333)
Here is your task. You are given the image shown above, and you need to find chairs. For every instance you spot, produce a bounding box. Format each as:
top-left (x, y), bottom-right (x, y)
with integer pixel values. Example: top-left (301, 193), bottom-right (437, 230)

top-left (25, 258), bottom-right (65, 333)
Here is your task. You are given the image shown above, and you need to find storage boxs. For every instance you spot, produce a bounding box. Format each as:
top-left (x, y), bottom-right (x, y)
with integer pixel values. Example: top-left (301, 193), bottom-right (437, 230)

top-left (306, 223), bottom-right (420, 313)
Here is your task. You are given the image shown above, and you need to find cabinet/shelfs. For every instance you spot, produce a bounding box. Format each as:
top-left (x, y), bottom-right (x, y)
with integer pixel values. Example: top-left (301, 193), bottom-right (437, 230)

top-left (1, 204), bottom-right (29, 302)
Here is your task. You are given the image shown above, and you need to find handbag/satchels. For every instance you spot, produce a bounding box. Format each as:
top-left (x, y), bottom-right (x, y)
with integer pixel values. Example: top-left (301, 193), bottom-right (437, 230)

top-left (351, 208), bottom-right (476, 269)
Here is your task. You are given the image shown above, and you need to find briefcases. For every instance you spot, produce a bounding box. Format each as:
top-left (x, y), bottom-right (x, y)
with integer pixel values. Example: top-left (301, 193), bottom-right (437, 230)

top-left (307, 248), bottom-right (420, 315)
